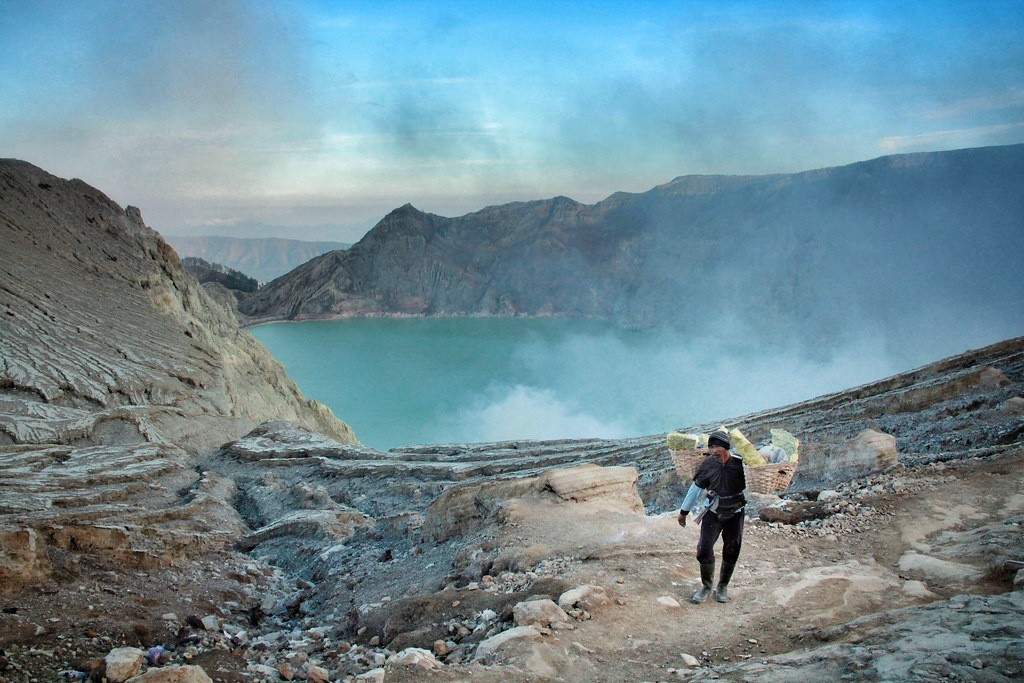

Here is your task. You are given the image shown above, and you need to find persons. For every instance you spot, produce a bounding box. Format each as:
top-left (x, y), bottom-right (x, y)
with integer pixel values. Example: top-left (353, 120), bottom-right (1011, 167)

top-left (677, 432), bottom-right (747, 604)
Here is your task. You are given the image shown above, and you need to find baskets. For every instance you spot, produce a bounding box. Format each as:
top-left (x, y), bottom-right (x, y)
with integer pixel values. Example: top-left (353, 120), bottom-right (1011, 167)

top-left (668, 447), bottom-right (710, 488)
top-left (742, 458), bottom-right (797, 495)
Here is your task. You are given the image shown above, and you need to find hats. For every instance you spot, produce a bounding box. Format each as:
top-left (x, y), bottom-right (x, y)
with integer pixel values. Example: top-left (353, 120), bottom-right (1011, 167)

top-left (708, 431), bottom-right (730, 449)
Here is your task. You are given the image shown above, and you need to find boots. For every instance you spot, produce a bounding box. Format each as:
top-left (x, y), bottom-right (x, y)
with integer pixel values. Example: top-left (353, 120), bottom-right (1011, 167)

top-left (716, 561), bottom-right (737, 603)
top-left (692, 561), bottom-right (715, 603)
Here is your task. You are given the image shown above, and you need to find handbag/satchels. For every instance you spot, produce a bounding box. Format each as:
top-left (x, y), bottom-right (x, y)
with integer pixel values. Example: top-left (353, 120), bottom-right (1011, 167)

top-left (710, 502), bottom-right (744, 521)
